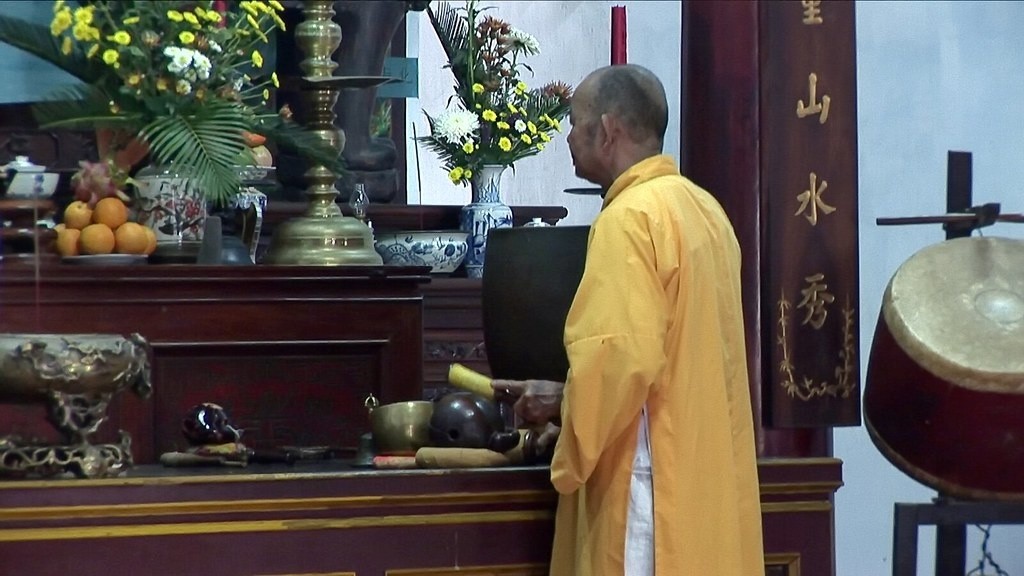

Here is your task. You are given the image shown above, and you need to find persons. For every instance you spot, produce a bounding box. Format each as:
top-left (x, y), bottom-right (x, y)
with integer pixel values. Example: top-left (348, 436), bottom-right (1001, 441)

top-left (490, 60), bottom-right (764, 576)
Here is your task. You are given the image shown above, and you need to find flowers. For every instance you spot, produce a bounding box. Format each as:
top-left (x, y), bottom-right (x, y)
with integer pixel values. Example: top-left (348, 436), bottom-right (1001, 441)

top-left (413, 0), bottom-right (573, 183)
top-left (0, 0), bottom-right (351, 178)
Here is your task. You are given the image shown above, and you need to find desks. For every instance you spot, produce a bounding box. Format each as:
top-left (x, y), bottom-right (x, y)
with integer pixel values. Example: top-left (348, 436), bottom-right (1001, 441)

top-left (0, 251), bottom-right (434, 455)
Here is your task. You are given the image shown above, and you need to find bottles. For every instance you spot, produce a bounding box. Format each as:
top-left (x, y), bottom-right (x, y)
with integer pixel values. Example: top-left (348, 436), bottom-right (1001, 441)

top-left (132, 152), bottom-right (208, 246)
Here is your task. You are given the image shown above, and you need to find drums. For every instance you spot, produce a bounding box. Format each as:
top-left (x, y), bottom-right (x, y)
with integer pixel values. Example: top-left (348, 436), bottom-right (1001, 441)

top-left (862, 233), bottom-right (1024, 500)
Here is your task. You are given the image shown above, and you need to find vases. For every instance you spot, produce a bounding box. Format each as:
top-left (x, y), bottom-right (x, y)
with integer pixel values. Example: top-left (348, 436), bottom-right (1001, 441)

top-left (459, 166), bottom-right (510, 280)
top-left (119, 179), bottom-right (219, 263)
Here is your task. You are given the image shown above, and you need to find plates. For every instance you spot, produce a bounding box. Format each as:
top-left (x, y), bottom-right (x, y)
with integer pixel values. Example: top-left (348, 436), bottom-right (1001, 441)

top-left (61, 253), bottom-right (149, 266)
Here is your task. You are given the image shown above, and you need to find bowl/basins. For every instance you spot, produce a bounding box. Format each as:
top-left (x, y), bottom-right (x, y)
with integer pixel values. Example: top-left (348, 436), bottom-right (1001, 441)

top-left (372, 229), bottom-right (471, 272)
top-left (0, 172), bottom-right (60, 200)
top-left (371, 399), bottom-right (435, 457)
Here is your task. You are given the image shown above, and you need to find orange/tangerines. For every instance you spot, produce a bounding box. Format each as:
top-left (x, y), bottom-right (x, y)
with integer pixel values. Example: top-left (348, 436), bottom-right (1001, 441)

top-left (49, 197), bottom-right (156, 256)
